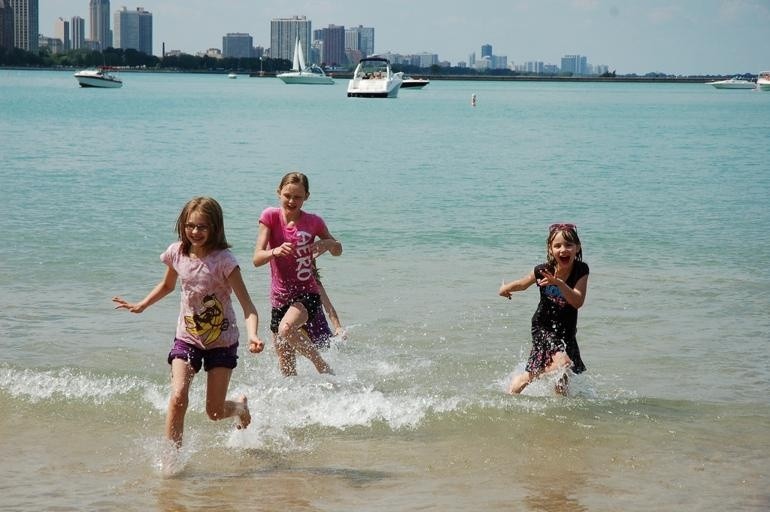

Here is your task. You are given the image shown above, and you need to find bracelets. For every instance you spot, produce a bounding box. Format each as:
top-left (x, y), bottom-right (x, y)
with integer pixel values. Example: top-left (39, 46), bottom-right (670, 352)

top-left (272, 247), bottom-right (275, 256)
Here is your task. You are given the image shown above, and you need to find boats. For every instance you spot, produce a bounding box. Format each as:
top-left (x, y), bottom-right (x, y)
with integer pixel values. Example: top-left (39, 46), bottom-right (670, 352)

top-left (757, 72), bottom-right (769, 90)
top-left (346, 54), bottom-right (403, 99)
top-left (275, 63), bottom-right (335, 84)
top-left (704, 77), bottom-right (756, 90)
top-left (75, 66), bottom-right (122, 88)
top-left (228, 71), bottom-right (240, 78)
top-left (400, 75), bottom-right (430, 90)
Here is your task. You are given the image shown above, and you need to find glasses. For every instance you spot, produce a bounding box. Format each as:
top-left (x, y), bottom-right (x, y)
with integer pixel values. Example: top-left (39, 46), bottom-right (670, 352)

top-left (183, 224), bottom-right (210, 231)
top-left (547, 222), bottom-right (578, 235)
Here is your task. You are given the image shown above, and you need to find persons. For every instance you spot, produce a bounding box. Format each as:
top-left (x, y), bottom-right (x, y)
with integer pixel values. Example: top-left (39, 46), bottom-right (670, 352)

top-left (112, 196), bottom-right (265, 449)
top-left (497, 223), bottom-right (590, 397)
top-left (252, 173), bottom-right (347, 377)
